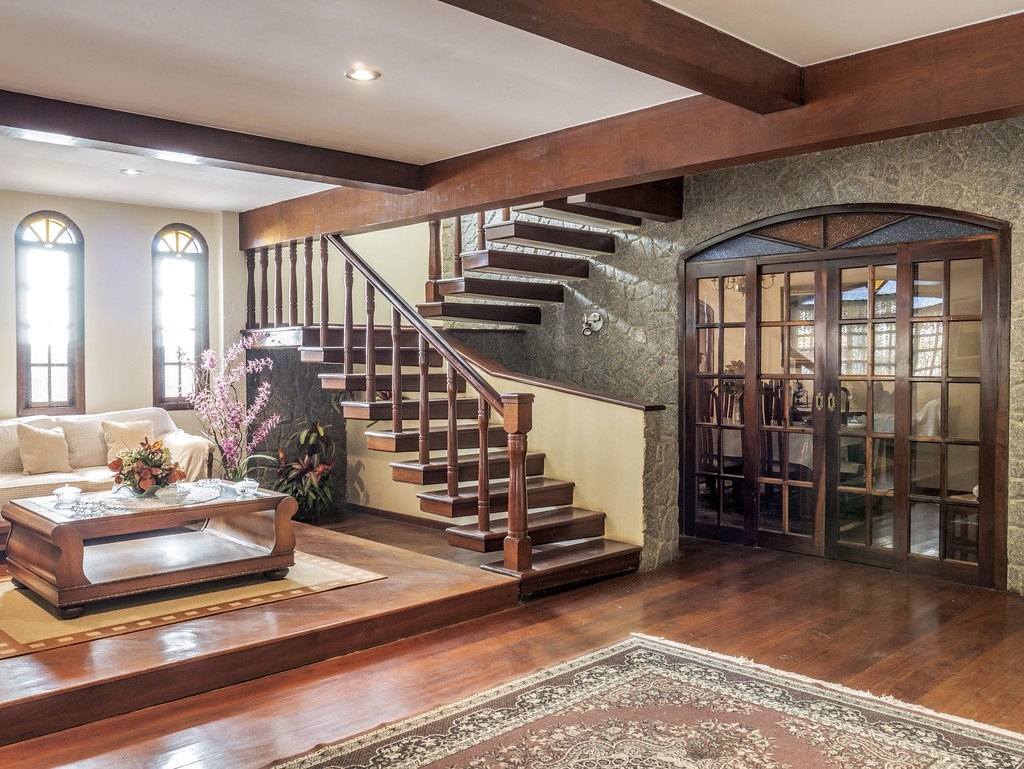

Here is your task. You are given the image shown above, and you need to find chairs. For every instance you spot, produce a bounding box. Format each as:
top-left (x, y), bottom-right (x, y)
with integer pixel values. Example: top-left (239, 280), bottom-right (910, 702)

top-left (699, 383), bottom-right (919, 519)
top-left (947, 492), bottom-right (980, 562)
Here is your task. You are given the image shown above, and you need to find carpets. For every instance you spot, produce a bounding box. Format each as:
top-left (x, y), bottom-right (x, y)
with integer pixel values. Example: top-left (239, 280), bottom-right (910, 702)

top-left (0, 526), bottom-right (389, 661)
top-left (254, 630), bottom-right (1024, 769)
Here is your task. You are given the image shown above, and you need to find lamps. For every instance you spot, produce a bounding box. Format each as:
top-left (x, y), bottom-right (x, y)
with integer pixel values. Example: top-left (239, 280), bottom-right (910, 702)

top-left (711, 274), bottom-right (778, 298)
top-left (582, 312), bottom-right (604, 336)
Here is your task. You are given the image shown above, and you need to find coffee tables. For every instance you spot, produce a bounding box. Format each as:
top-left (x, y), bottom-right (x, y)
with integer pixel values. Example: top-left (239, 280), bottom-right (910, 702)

top-left (0, 477), bottom-right (298, 621)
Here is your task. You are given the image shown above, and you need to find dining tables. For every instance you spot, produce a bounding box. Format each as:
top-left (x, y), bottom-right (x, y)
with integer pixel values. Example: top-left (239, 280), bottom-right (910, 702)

top-left (703, 416), bottom-right (896, 518)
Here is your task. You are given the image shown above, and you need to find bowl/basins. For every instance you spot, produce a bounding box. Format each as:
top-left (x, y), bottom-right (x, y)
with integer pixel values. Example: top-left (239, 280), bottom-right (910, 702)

top-left (155, 484), bottom-right (190, 504)
top-left (53, 483), bottom-right (81, 503)
top-left (234, 477), bottom-right (258, 497)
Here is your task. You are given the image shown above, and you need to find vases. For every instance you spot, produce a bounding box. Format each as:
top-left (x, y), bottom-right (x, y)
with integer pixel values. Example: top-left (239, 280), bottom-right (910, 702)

top-left (733, 389), bottom-right (744, 423)
top-left (111, 484), bottom-right (177, 499)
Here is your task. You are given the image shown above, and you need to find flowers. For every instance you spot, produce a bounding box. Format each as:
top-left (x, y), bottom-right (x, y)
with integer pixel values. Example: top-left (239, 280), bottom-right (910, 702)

top-left (724, 360), bottom-right (745, 398)
top-left (177, 331), bottom-right (284, 482)
top-left (108, 435), bottom-right (186, 494)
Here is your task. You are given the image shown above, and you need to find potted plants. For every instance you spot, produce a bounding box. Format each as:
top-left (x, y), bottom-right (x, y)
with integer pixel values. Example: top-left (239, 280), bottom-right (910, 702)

top-left (269, 418), bottom-right (338, 526)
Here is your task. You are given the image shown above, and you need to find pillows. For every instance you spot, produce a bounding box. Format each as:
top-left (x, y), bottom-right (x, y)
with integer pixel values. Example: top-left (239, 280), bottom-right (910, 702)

top-left (101, 420), bottom-right (156, 464)
top-left (17, 423), bottom-right (75, 477)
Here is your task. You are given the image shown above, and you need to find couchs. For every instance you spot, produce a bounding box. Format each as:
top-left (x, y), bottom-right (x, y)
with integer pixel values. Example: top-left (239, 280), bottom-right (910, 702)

top-left (0, 405), bottom-right (215, 552)
